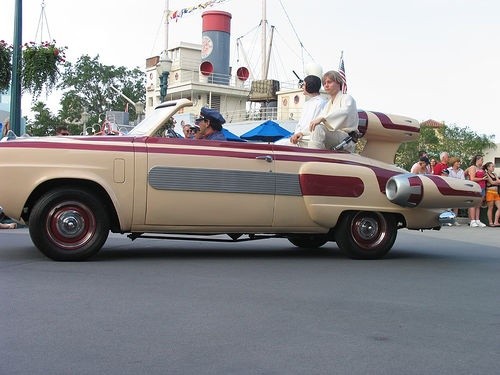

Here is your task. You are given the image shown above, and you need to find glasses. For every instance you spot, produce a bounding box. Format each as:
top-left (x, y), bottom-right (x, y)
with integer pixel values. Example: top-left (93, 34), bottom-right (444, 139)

top-left (186, 130), bottom-right (192, 132)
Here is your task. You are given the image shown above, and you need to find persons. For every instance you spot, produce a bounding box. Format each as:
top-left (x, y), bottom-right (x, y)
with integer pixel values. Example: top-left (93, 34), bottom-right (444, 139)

top-left (482, 162), bottom-right (500, 227)
top-left (55, 127), bottom-right (69, 135)
top-left (290, 71), bottom-right (359, 153)
top-left (195, 107), bottom-right (227, 141)
top-left (183, 124), bottom-right (201, 138)
top-left (274, 75), bottom-right (329, 146)
top-left (411, 151), bottom-right (438, 174)
top-left (433, 152), bottom-right (449, 175)
top-left (469, 155), bottom-right (487, 228)
top-left (447, 158), bottom-right (465, 226)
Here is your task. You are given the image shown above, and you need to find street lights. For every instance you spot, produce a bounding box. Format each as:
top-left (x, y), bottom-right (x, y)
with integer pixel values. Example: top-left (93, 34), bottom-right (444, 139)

top-left (156, 54), bottom-right (172, 102)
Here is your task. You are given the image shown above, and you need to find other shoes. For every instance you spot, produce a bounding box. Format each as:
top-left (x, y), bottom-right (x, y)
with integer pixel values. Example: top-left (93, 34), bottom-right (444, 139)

top-left (476, 220), bottom-right (486, 227)
top-left (470, 220), bottom-right (478, 227)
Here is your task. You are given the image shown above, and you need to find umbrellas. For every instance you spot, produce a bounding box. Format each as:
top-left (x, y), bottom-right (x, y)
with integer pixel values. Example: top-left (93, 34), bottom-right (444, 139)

top-left (240, 120), bottom-right (295, 144)
top-left (221, 128), bottom-right (247, 142)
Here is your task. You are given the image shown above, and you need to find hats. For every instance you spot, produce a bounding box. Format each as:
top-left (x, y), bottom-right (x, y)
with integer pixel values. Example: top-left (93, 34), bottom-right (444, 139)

top-left (419, 157), bottom-right (431, 164)
top-left (441, 169), bottom-right (449, 175)
top-left (195, 107), bottom-right (226, 124)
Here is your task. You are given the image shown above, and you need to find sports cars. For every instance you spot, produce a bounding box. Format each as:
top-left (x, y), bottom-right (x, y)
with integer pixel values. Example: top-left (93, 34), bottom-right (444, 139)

top-left (0, 99), bottom-right (483, 260)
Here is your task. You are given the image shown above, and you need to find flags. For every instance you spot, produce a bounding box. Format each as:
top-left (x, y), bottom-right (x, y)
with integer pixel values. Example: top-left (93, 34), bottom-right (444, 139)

top-left (339, 60), bottom-right (347, 94)
